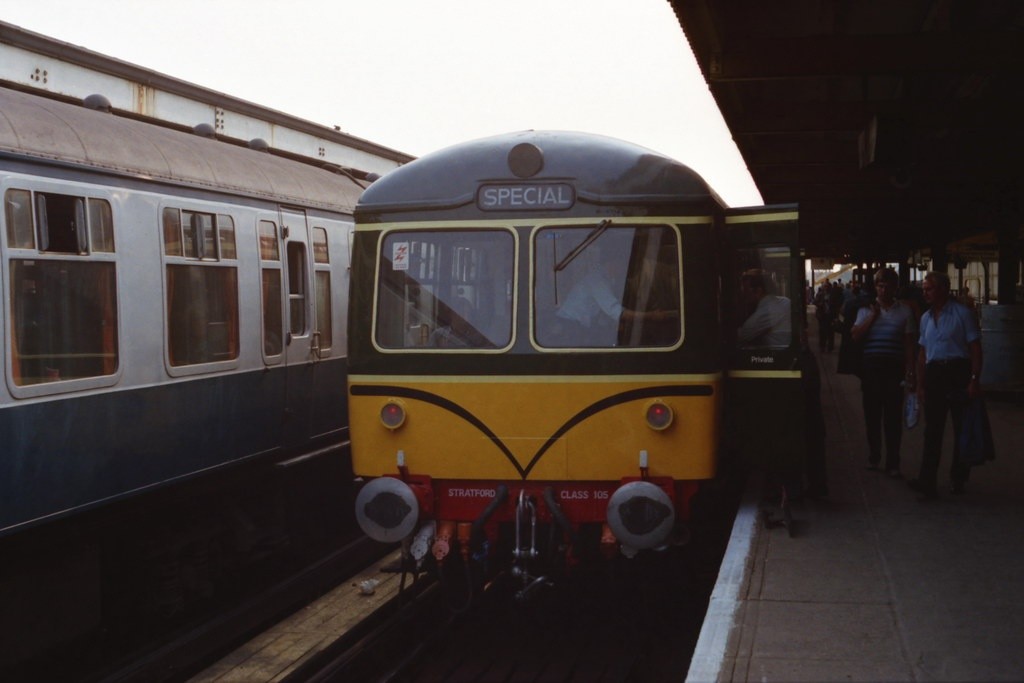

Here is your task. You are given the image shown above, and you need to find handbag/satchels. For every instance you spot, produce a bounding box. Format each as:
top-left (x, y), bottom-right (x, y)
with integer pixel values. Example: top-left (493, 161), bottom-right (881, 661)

top-left (904, 393), bottom-right (920, 430)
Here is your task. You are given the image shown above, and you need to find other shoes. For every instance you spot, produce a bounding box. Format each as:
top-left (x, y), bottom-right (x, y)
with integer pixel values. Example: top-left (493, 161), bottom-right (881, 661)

top-left (804, 484), bottom-right (827, 497)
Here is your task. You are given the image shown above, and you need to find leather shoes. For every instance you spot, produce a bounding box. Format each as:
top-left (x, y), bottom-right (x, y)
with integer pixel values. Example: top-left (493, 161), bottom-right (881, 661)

top-left (868, 461), bottom-right (878, 470)
top-left (908, 478), bottom-right (936, 492)
top-left (886, 464), bottom-right (899, 476)
top-left (950, 480), bottom-right (967, 495)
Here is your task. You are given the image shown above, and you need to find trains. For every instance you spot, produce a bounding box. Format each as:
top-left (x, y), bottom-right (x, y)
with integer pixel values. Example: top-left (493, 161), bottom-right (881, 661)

top-left (345, 129), bottom-right (807, 605)
top-left (0, 78), bottom-right (471, 539)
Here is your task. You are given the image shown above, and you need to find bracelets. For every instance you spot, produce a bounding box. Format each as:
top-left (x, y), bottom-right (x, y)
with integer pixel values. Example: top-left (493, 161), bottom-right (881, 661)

top-left (971, 374), bottom-right (978, 380)
top-left (905, 368), bottom-right (913, 376)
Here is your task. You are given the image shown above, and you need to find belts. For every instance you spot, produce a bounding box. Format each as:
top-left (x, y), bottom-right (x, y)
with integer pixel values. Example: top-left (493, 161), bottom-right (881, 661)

top-left (925, 360), bottom-right (965, 366)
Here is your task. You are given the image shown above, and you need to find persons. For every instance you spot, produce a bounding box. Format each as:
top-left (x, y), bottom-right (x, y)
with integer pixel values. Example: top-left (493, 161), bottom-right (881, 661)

top-left (550, 245), bottom-right (671, 336)
top-left (959, 286), bottom-right (975, 310)
top-left (850, 269), bottom-right (917, 479)
top-left (736, 271), bottom-right (830, 501)
top-left (814, 277), bottom-right (861, 352)
top-left (902, 272), bottom-right (983, 497)
top-left (425, 297), bottom-right (489, 348)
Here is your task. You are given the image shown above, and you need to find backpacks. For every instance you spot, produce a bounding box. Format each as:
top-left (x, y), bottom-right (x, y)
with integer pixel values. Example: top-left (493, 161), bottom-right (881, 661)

top-left (842, 307), bottom-right (864, 353)
